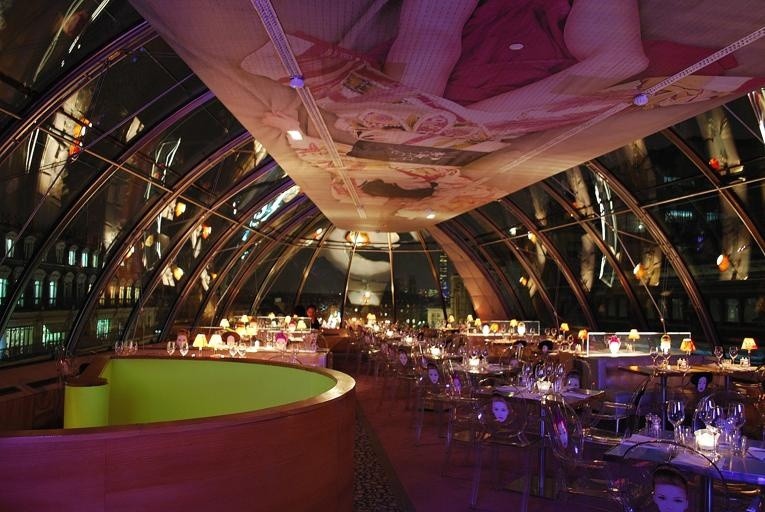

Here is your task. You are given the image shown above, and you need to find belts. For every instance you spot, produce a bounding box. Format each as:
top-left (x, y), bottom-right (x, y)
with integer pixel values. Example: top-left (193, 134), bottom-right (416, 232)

top-left (714, 346), bottom-right (738, 369)
top-left (649, 347), bottom-right (672, 373)
top-left (361, 319), bottom-right (535, 369)
top-left (168, 321), bottom-right (320, 358)
top-left (519, 349), bottom-right (565, 398)
top-left (665, 397), bottom-right (746, 457)
top-left (544, 327), bottom-right (574, 353)
top-left (114, 340), bottom-right (138, 356)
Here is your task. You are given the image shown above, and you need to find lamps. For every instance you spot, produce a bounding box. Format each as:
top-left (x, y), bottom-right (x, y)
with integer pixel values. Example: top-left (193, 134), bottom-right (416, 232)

top-left (171, 201), bottom-right (187, 219)
top-left (518, 275), bottom-right (527, 287)
top-left (633, 263), bottom-right (646, 280)
top-left (716, 253), bottom-right (731, 273)
top-left (67, 117), bottom-right (93, 157)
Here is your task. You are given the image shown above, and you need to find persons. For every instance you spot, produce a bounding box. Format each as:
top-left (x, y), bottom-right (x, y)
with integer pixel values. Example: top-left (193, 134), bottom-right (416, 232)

top-left (293, 0), bottom-right (650, 199)
top-left (652, 470), bottom-right (689, 512)
top-left (274, 333), bottom-right (286, 350)
top-left (176, 329), bottom-right (188, 347)
top-left (698, 377), bottom-right (706, 392)
top-left (222, 331), bottom-right (240, 346)
top-left (558, 422), bottom-right (568, 447)
top-left (567, 371), bottom-right (580, 388)
top-left (305, 303), bottom-right (320, 330)
top-left (453, 377), bottom-right (461, 392)
top-left (398, 349), bottom-right (408, 366)
top-left (492, 399), bottom-right (509, 423)
top-left (509, 356), bottom-right (519, 368)
top-left (427, 363), bottom-right (439, 384)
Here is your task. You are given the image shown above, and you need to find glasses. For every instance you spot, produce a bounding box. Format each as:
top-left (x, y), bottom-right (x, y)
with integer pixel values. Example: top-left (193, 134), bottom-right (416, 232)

top-left (633, 263), bottom-right (646, 280)
top-left (716, 253), bottom-right (731, 273)
top-left (67, 117), bottom-right (93, 157)
top-left (171, 201), bottom-right (187, 219)
top-left (518, 275), bottom-right (527, 287)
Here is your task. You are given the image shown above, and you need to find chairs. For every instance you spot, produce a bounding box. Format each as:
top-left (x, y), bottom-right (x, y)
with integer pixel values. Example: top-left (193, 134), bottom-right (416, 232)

top-left (190, 308), bottom-right (765, 512)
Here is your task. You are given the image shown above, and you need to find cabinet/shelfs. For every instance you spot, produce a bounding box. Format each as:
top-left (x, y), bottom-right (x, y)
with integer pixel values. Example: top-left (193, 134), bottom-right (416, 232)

top-left (645, 413), bottom-right (660, 426)
top-left (739, 356), bottom-right (751, 369)
top-left (607, 336), bottom-right (621, 354)
top-left (575, 344), bottom-right (582, 353)
top-left (677, 358), bottom-right (687, 368)
top-left (335, 319), bottom-right (340, 330)
